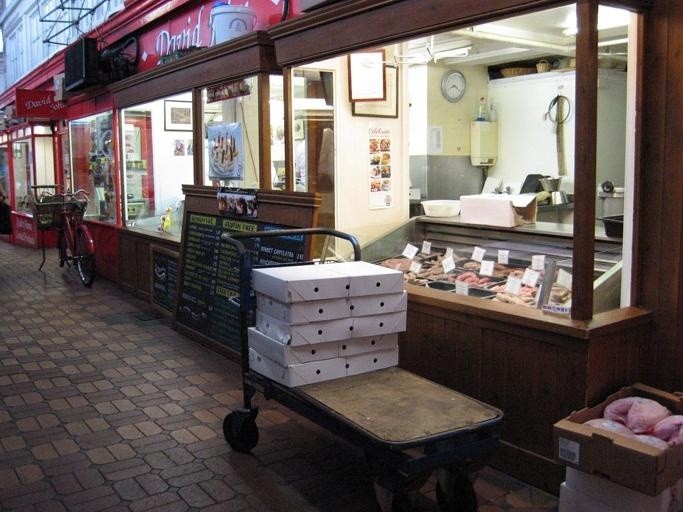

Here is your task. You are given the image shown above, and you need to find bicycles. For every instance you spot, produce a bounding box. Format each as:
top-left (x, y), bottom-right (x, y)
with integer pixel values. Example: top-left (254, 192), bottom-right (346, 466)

top-left (47, 188), bottom-right (98, 287)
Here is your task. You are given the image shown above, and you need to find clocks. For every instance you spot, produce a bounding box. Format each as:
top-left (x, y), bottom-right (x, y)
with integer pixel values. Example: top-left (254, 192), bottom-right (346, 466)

top-left (440, 67), bottom-right (468, 103)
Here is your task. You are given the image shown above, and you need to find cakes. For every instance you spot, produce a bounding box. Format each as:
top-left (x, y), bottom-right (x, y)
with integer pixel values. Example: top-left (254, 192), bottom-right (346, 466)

top-left (211, 139), bottom-right (238, 172)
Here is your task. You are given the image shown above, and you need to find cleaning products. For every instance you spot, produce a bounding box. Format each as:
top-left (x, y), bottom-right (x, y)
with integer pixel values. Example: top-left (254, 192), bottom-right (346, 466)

top-left (489, 97), bottom-right (496, 120)
top-left (478, 94), bottom-right (485, 121)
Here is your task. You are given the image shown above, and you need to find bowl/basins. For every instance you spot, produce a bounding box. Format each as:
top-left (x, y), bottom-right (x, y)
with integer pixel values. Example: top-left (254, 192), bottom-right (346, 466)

top-left (537, 177), bottom-right (561, 192)
top-left (549, 191), bottom-right (571, 205)
top-left (420, 199), bottom-right (460, 217)
top-left (127, 194), bottom-right (133, 199)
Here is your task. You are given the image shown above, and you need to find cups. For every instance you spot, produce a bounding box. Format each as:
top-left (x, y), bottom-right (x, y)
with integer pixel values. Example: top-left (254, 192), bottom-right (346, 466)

top-left (382, 181), bottom-right (390, 187)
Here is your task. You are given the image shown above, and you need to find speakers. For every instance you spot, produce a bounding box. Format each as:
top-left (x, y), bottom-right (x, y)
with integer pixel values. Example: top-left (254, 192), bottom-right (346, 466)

top-left (64, 37), bottom-right (98, 92)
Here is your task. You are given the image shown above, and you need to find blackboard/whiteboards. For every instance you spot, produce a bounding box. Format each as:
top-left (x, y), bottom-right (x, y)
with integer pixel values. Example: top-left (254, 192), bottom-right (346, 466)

top-left (149, 184), bottom-right (321, 367)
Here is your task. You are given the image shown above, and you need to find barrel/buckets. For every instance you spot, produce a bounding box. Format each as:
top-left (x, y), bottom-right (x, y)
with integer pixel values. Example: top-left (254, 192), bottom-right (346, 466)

top-left (551, 191), bottom-right (569, 207)
top-left (537, 176), bottom-right (562, 193)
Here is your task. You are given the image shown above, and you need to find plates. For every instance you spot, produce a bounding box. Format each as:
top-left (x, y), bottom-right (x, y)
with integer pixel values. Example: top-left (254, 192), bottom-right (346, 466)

top-left (381, 186), bottom-right (391, 191)
top-left (370, 170), bottom-right (379, 178)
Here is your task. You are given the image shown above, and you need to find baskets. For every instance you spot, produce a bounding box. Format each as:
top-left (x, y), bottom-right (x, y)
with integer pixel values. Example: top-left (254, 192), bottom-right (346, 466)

top-left (32, 193), bottom-right (86, 230)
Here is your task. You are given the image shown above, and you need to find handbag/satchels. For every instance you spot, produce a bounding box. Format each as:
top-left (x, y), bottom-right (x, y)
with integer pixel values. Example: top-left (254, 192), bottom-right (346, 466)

top-left (0, 196), bottom-right (13, 235)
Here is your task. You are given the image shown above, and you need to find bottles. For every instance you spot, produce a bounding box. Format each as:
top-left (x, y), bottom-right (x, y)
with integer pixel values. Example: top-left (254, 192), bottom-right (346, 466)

top-left (125, 159), bottom-right (147, 170)
top-left (16, 195), bottom-right (30, 210)
top-left (487, 104), bottom-right (495, 122)
top-left (161, 199), bottom-right (183, 230)
top-left (476, 97), bottom-right (486, 121)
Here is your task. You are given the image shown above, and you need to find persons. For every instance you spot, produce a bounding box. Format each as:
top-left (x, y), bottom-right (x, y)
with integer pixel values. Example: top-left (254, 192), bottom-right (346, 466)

top-left (94, 164), bottom-right (104, 179)
top-left (218, 196), bottom-right (257, 217)
top-left (0, 183), bottom-right (9, 199)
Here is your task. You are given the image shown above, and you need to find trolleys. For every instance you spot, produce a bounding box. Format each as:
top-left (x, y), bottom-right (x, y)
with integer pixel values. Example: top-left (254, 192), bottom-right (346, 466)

top-left (217, 228), bottom-right (506, 512)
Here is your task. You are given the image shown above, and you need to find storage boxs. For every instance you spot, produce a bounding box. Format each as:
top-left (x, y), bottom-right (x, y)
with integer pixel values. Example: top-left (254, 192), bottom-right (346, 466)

top-left (564, 465), bottom-right (682, 512)
top-left (459, 191), bottom-right (537, 230)
top-left (244, 258), bottom-right (407, 388)
top-left (594, 214), bottom-right (622, 238)
top-left (548, 381), bottom-right (682, 496)
top-left (557, 479), bottom-right (617, 512)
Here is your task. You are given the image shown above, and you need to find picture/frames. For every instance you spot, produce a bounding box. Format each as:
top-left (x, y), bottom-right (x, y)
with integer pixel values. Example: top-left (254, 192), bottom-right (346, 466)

top-left (162, 99), bottom-right (193, 132)
top-left (293, 107), bottom-right (306, 142)
top-left (351, 61), bottom-right (401, 120)
top-left (345, 47), bottom-right (388, 103)
